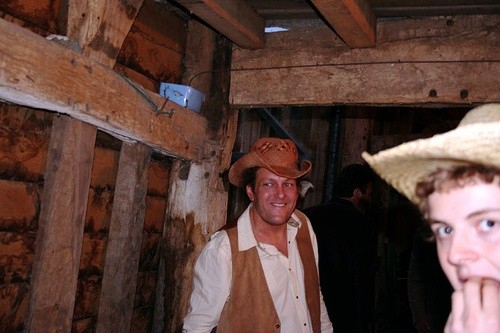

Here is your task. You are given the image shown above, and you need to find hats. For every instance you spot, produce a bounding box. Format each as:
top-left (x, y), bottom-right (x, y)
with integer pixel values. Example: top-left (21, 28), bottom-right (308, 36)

top-left (362, 103), bottom-right (500, 204)
top-left (228, 137), bottom-right (312, 187)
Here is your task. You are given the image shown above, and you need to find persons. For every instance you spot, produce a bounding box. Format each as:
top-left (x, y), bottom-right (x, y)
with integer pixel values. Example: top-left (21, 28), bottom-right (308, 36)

top-left (181, 137), bottom-right (333, 333)
top-left (302, 164), bottom-right (379, 333)
top-left (361, 103), bottom-right (500, 333)
